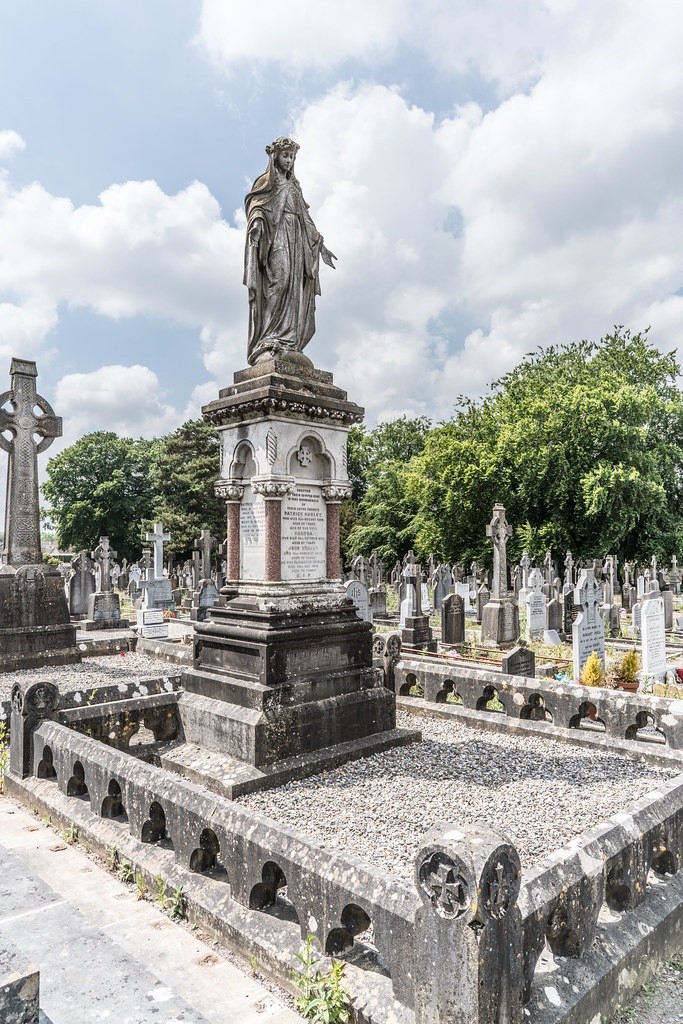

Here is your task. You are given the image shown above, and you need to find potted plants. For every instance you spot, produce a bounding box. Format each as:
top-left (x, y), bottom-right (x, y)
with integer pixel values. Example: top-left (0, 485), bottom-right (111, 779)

top-left (617, 649), bottom-right (642, 694)
top-left (581, 649), bottom-right (606, 720)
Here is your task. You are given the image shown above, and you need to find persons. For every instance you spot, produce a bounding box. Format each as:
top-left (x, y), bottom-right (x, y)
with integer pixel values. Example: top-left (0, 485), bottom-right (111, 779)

top-left (242, 137), bottom-right (338, 367)
